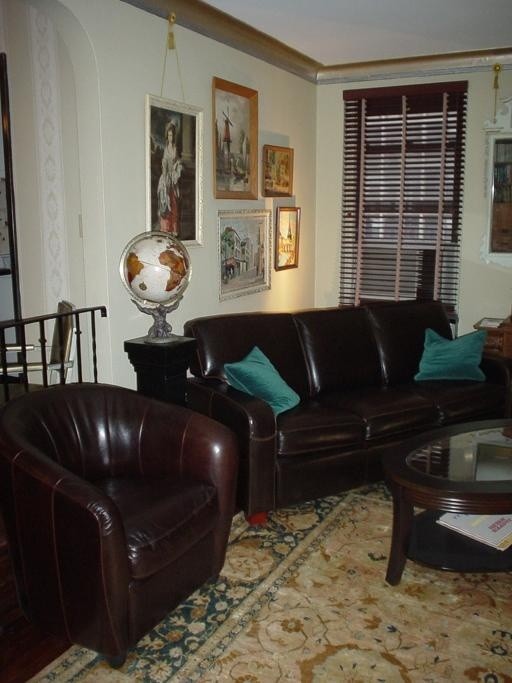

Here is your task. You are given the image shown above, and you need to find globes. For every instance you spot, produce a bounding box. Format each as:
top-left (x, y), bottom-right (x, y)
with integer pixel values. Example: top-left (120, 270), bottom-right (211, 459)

top-left (119, 232), bottom-right (193, 344)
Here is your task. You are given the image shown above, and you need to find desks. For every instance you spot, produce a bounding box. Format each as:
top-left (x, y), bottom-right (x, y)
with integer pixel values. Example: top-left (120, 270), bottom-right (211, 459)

top-left (475, 315), bottom-right (511, 357)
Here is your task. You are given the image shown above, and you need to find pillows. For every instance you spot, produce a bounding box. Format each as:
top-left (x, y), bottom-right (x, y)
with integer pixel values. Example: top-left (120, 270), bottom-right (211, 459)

top-left (226, 341), bottom-right (298, 418)
top-left (415, 322), bottom-right (485, 384)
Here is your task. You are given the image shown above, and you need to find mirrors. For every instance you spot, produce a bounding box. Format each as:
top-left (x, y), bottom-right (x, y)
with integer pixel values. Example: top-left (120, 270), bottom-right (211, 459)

top-left (481, 95), bottom-right (512, 264)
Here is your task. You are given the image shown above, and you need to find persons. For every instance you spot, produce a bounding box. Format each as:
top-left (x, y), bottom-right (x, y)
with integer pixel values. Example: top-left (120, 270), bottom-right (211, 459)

top-left (157, 123), bottom-right (185, 240)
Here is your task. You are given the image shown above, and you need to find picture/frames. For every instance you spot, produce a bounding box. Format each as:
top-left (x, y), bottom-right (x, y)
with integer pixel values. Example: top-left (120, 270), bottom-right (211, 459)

top-left (210, 75), bottom-right (261, 200)
top-left (273, 206), bottom-right (300, 271)
top-left (144, 91), bottom-right (204, 245)
top-left (262, 144), bottom-right (294, 195)
top-left (216, 208), bottom-right (272, 301)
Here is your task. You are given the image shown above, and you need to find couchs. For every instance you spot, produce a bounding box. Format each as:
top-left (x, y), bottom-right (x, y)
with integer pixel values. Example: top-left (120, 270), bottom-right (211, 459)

top-left (182, 299), bottom-right (491, 520)
top-left (2, 380), bottom-right (238, 673)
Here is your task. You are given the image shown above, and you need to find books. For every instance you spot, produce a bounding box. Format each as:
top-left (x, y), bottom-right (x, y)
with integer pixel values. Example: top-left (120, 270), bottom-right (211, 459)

top-left (435, 512), bottom-right (512, 551)
